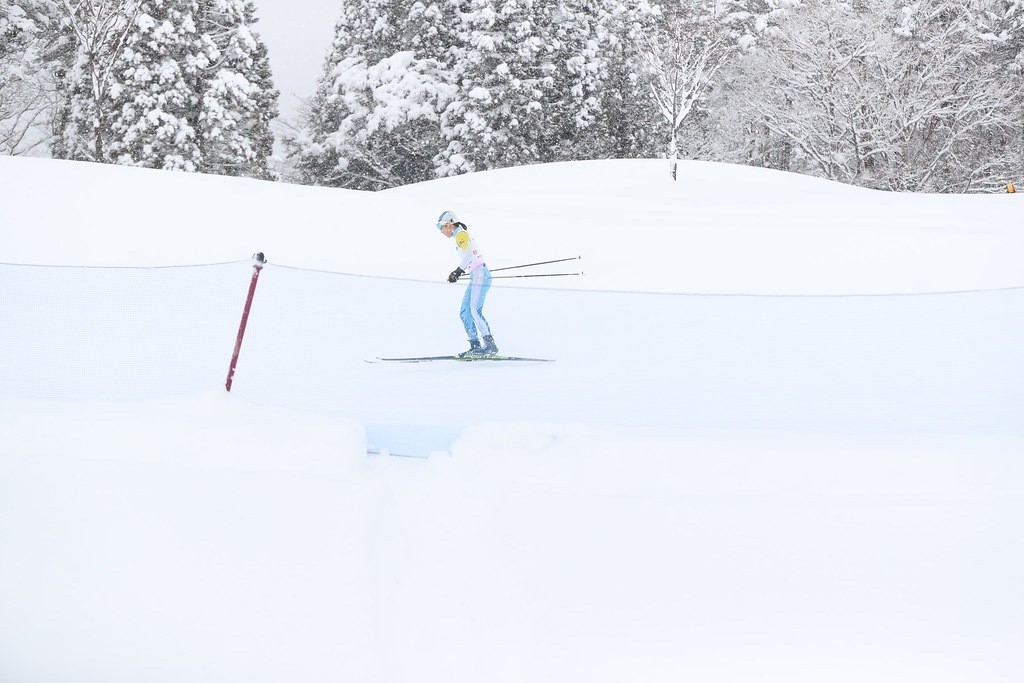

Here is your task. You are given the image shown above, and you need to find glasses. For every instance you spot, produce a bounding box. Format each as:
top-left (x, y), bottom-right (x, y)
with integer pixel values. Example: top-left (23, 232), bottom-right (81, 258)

top-left (436, 224), bottom-right (441, 230)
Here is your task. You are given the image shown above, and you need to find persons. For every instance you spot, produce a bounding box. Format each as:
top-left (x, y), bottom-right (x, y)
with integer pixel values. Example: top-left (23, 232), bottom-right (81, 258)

top-left (437, 210), bottom-right (498, 357)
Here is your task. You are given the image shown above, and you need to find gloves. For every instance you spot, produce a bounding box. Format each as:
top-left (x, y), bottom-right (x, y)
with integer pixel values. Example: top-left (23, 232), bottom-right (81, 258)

top-left (449, 267), bottom-right (465, 283)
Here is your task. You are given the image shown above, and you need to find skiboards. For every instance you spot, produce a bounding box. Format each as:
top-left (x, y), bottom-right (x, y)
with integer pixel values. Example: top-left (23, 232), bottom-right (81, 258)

top-left (363, 354), bottom-right (556, 363)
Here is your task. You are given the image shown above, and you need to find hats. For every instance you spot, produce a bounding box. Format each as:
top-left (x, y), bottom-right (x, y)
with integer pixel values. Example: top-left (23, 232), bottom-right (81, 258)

top-left (437, 211), bottom-right (458, 226)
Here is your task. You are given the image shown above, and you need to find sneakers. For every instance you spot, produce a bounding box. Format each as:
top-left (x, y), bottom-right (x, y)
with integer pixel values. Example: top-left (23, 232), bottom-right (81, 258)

top-left (479, 335), bottom-right (498, 355)
top-left (463, 339), bottom-right (484, 355)
